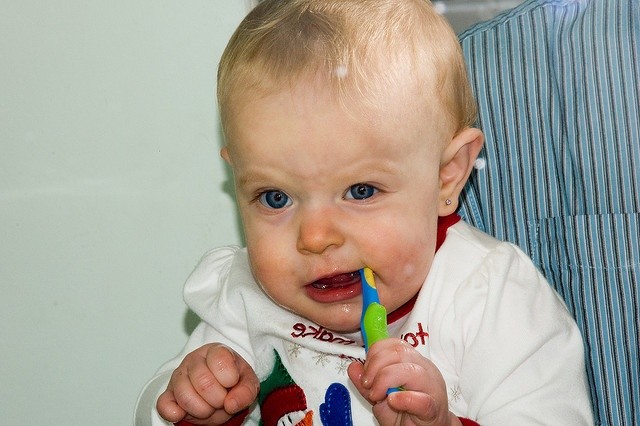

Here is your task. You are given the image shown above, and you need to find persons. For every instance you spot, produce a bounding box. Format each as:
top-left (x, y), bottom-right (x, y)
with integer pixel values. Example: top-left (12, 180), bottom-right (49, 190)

top-left (133, 0), bottom-right (595, 426)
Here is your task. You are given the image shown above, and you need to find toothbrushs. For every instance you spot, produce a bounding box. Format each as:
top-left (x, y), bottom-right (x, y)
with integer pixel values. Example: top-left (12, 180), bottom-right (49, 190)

top-left (358, 267), bottom-right (401, 396)
